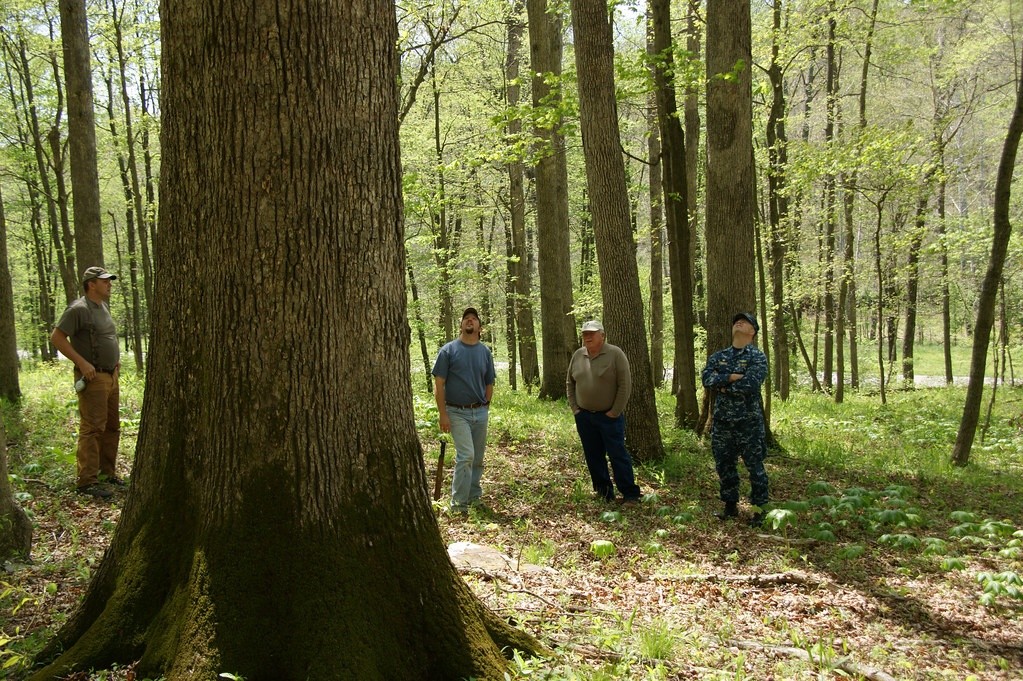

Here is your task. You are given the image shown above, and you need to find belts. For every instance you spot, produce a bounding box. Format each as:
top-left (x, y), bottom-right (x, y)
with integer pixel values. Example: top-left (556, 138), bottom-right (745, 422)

top-left (579, 408), bottom-right (611, 413)
top-left (74, 366), bottom-right (114, 374)
top-left (446, 402), bottom-right (484, 408)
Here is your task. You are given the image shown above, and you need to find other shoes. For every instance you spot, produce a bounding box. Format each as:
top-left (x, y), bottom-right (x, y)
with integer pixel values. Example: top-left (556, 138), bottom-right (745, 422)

top-left (713, 511), bottom-right (738, 521)
top-left (749, 510), bottom-right (766, 526)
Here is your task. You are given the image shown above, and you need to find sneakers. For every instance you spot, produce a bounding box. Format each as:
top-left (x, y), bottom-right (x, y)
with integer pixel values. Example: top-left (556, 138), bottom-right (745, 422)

top-left (99, 474), bottom-right (128, 489)
top-left (77, 485), bottom-right (114, 497)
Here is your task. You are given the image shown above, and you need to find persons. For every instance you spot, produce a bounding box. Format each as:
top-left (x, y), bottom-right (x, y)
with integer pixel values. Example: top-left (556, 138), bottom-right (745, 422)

top-left (700, 312), bottom-right (770, 526)
top-left (50, 266), bottom-right (126, 497)
top-left (566, 321), bottom-right (641, 506)
top-left (431, 309), bottom-right (499, 522)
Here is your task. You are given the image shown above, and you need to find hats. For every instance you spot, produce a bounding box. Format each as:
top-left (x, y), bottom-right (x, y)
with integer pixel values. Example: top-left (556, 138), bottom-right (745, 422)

top-left (82, 266), bottom-right (117, 280)
top-left (463, 308), bottom-right (481, 321)
top-left (582, 321), bottom-right (604, 331)
top-left (733, 312), bottom-right (759, 335)
